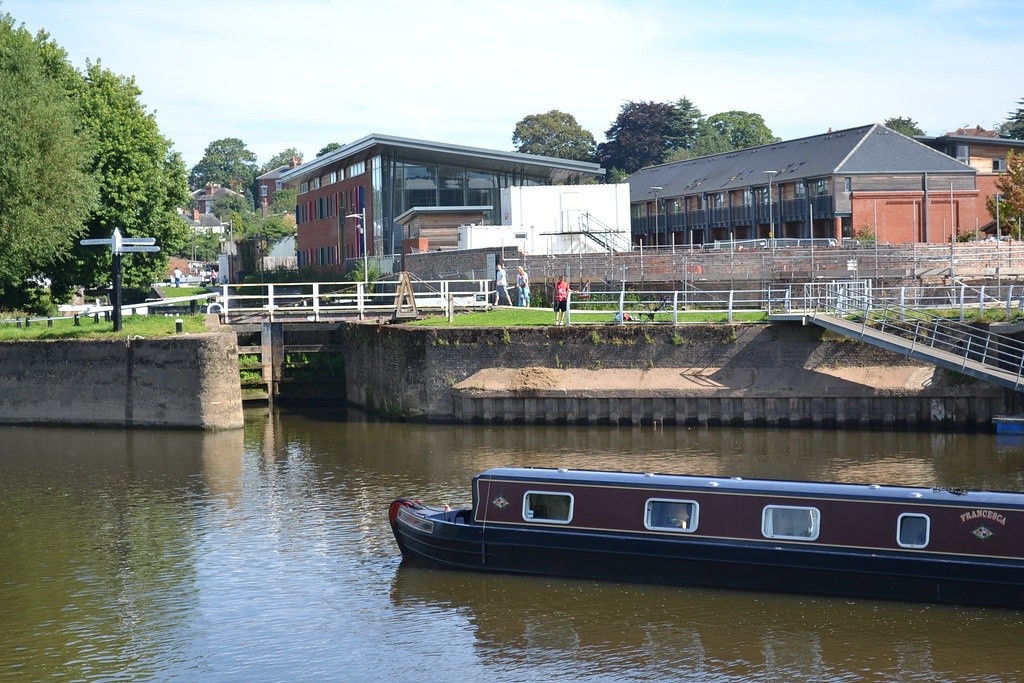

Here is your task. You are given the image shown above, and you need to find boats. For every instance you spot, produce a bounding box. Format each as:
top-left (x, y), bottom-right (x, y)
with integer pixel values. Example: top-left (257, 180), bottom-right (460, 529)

top-left (387, 465), bottom-right (1024, 611)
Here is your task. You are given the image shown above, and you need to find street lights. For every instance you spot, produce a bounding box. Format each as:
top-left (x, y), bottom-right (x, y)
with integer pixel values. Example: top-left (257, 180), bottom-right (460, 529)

top-left (220, 220), bottom-right (233, 285)
top-left (650, 186), bottom-right (664, 250)
top-left (762, 170), bottom-right (778, 249)
top-left (345, 207), bottom-right (368, 289)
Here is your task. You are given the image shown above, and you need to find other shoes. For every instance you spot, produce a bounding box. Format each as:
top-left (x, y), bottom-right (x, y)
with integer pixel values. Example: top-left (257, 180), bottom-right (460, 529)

top-left (555, 320), bottom-right (558, 325)
top-left (560, 321), bottom-right (563, 325)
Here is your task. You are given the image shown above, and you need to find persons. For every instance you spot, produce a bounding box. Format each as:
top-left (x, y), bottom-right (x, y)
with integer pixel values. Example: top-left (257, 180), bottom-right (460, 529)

top-left (173, 267), bottom-right (183, 288)
top-left (493, 263), bottom-right (513, 306)
top-left (516, 265), bottom-right (531, 308)
top-left (553, 275), bottom-right (576, 326)
top-left (210, 269), bottom-right (217, 286)
